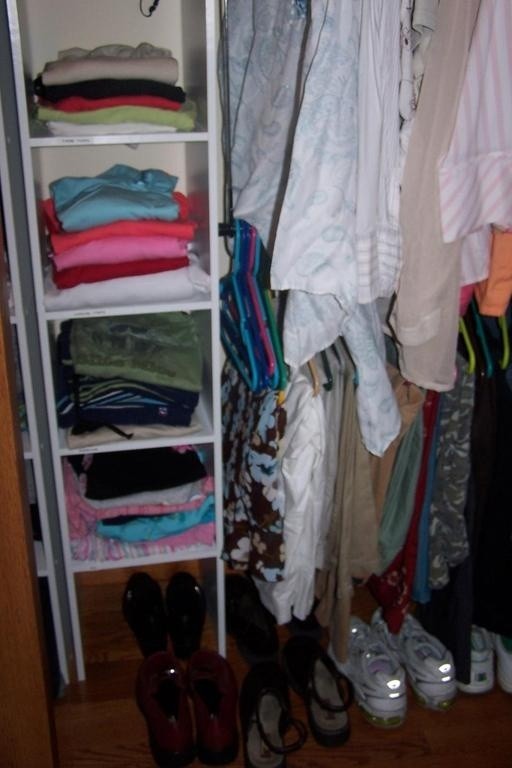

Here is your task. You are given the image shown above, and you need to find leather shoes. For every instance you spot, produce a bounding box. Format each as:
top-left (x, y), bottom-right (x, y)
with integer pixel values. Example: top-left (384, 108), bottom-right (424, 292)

top-left (122, 571), bottom-right (238, 768)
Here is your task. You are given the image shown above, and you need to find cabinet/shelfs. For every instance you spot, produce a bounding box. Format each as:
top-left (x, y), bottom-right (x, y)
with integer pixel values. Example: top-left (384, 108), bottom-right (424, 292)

top-left (7, 0), bottom-right (229, 684)
top-left (2, 95), bottom-right (73, 686)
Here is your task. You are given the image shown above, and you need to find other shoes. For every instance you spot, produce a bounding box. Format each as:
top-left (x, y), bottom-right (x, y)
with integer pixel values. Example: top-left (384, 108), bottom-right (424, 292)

top-left (226, 573), bottom-right (280, 661)
top-left (285, 595), bottom-right (323, 639)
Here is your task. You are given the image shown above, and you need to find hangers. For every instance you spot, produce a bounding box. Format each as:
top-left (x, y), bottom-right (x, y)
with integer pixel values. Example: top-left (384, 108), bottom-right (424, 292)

top-left (218, 217), bottom-right (511, 394)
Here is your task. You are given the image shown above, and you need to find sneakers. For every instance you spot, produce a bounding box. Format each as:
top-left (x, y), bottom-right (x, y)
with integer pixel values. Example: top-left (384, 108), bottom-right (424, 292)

top-left (390, 612), bottom-right (457, 708)
top-left (491, 629), bottom-right (512, 693)
top-left (454, 625), bottom-right (494, 693)
top-left (327, 615), bottom-right (407, 729)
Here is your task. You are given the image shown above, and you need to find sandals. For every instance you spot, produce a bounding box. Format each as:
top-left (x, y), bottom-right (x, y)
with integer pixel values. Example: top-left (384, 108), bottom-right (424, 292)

top-left (283, 632), bottom-right (356, 747)
top-left (241, 663), bottom-right (309, 767)
